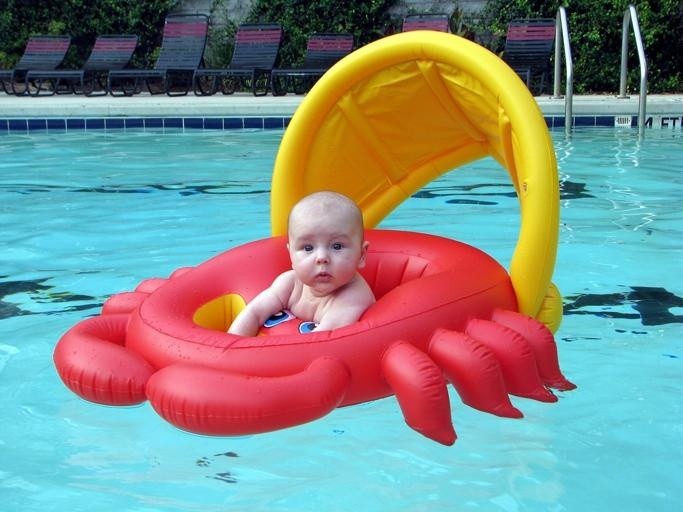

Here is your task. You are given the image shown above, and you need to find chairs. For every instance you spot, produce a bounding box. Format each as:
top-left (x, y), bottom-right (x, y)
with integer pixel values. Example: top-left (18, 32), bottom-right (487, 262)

top-left (269, 34), bottom-right (354, 94)
top-left (189, 23), bottom-right (283, 95)
top-left (400, 12), bottom-right (451, 31)
top-left (108, 15), bottom-right (212, 96)
top-left (25, 34), bottom-right (138, 97)
top-left (0, 33), bottom-right (71, 95)
top-left (496, 19), bottom-right (555, 98)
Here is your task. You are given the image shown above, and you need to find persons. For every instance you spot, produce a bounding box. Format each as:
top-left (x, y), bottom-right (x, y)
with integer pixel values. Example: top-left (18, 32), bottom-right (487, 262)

top-left (224, 190), bottom-right (377, 337)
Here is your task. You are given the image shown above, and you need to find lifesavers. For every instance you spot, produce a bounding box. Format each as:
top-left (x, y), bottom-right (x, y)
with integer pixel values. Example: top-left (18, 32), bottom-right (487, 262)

top-left (53, 29), bottom-right (577, 446)
